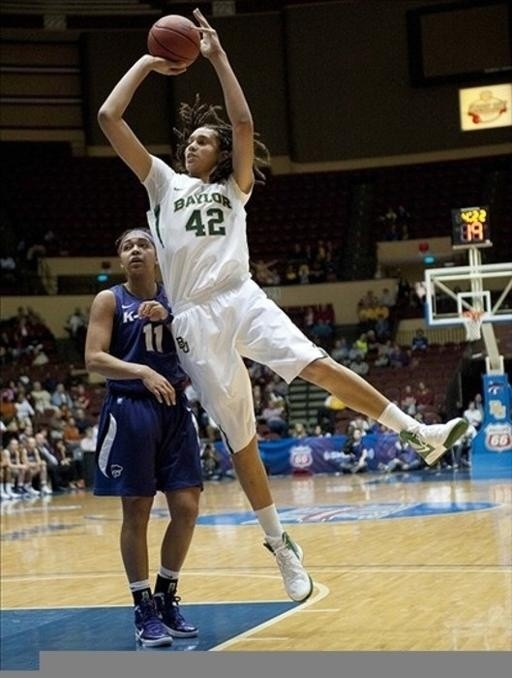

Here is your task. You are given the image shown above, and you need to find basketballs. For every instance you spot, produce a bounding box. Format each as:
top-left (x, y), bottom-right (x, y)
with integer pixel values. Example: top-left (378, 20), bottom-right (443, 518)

top-left (148, 15), bottom-right (201, 70)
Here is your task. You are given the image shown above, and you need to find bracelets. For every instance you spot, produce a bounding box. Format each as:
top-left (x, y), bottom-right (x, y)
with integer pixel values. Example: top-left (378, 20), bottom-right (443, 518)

top-left (161, 313), bottom-right (174, 326)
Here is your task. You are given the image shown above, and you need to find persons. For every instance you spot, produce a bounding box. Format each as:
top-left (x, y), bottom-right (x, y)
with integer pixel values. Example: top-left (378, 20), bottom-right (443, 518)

top-left (97, 6), bottom-right (471, 602)
top-left (18, 230), bottom-right (68, 266)
top-left (2, 305), bottom-right (102, 501)
top-left (83, 228), bottom-right (204, 646)
top-left (200, 202), bottom-right (482, 481)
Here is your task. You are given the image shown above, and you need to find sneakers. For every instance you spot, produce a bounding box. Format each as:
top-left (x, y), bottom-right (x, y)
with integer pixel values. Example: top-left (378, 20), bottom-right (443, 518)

top-left (0, 485), bottom-right (53, 500)
top-left (151, 591), bottom-right (199, 638)
top-left (133, 599), bottom-right (173, 647)
top-left (398, 417), bottom-right (469, 467)
top-left (263, 529), bottom-right (313, 603)
